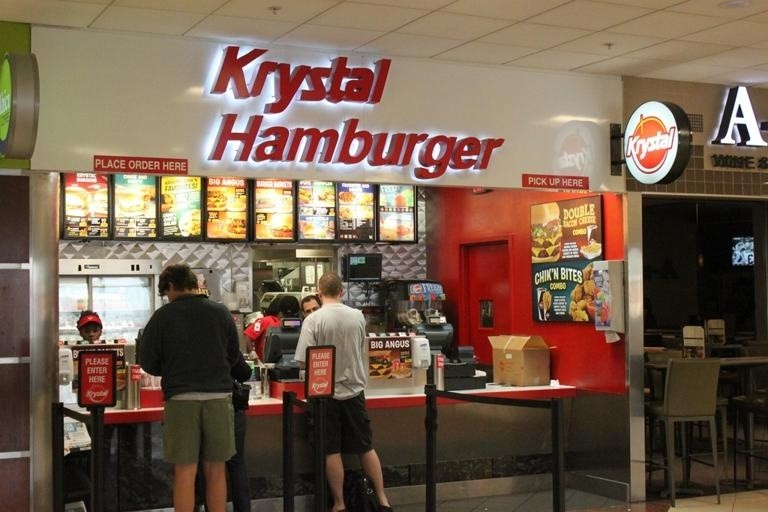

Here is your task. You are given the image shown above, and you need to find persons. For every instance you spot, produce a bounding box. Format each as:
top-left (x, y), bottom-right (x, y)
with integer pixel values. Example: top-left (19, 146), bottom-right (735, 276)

top-left (137, 263), bottom-right (243, 512)
top-left (300, 295), bottom-right (321, 319)
top-left (242, 295), bottom-right (282, 382)
top-left (77, 310), bottom-right (105, 342)
top-left (193, 353), bottom-right (259, 512)
top-left (293, 272), bottom-right (395, 512)
top-left (278, 295), bottom-right (301, 319)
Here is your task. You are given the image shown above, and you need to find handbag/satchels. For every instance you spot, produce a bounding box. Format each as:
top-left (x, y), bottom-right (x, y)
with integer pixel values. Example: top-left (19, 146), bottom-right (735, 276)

top-left (232, 382), bottom-right (251, 409)
top-left (343, 469), bottom-right (379, 512)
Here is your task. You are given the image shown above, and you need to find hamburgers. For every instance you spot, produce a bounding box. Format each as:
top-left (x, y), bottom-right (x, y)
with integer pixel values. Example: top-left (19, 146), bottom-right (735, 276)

top-left (206, 187), bottom-right (230, 212)
top-left (532, 203), bottom-right (562, 263)
top-left (224, 214), bottom-right (248, 238)
top-left (66, 186), bottom-right (149, 217)
top-left (269, 213), bottom-right (293, 237)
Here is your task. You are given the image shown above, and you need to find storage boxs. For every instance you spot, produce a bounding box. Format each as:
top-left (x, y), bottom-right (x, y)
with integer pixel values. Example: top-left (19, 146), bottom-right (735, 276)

top-left (487, 333), bottom-right (556, 386)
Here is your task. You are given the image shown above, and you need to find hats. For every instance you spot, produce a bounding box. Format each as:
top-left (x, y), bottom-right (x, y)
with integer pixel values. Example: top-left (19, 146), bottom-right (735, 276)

top-left (77, 314), bottom-right (102, 328)
top-left (279, 296), bottom-right (299, 314)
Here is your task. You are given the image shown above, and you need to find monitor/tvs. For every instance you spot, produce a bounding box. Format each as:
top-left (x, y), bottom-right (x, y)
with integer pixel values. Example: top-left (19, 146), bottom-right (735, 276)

top-left (347, 253), bottom-right (382, 281)
top-left (262, 326), bottom-right (301, 363)
top-left (415, 323), bottom-right (454, 354)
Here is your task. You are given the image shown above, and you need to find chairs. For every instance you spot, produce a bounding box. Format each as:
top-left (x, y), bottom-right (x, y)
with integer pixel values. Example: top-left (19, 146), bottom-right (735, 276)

top-left (643, 344), bottom-right (767, 509)
